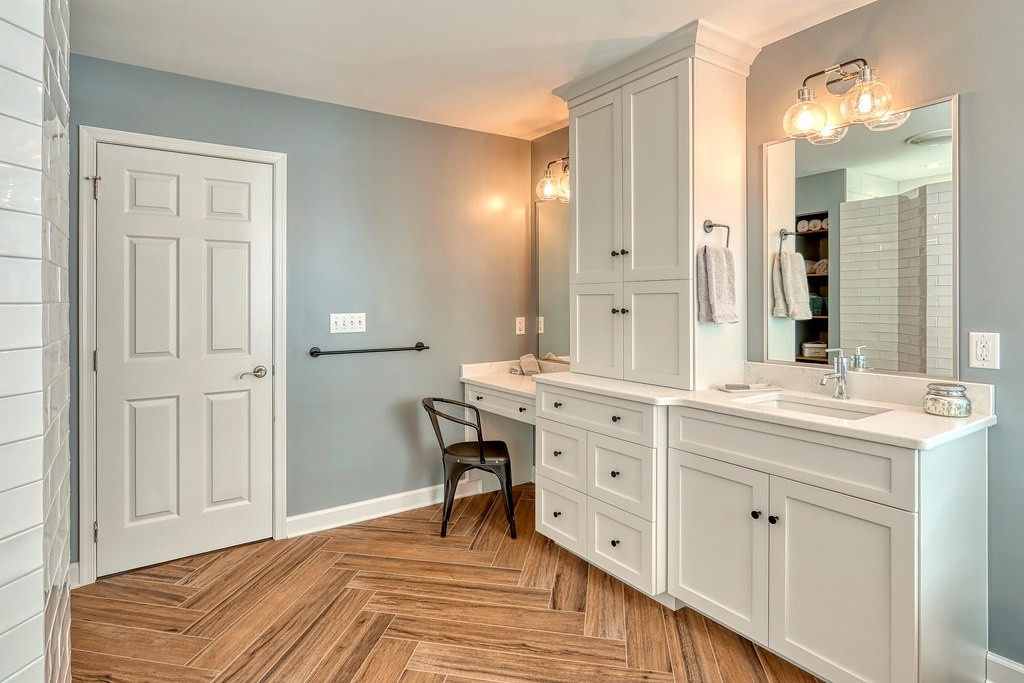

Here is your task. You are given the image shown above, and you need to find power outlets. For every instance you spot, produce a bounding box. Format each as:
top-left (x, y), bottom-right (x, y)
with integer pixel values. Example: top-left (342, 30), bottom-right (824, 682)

top-left (330, 313), bottom-right (366, 333)
top-left (968, 331), bottom-right (1001, 370)
top-left (515, 317), bottom-right (526, 336)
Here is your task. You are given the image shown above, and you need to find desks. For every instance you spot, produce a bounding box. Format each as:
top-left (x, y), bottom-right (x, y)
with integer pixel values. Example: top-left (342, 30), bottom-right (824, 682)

top-left (460, 357), bottom-right (570, 491)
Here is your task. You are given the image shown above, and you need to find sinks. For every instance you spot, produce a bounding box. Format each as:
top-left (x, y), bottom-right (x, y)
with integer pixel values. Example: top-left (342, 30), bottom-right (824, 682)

top-left (731, 392), bottom-right (897, 421)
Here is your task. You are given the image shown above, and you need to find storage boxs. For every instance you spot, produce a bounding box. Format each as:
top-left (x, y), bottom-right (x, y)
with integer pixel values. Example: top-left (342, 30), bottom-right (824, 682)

top-left (801, 341), bottom-right (827, 357)
top-left (809, 298), bottom-right (824, 315)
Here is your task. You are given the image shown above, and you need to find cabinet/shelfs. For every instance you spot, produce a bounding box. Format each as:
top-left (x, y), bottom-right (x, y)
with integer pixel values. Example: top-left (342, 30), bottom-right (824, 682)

top-left (531, 371), bottom-right (687, 613)
top-left (669, 362), bottom-right (998, 683)
top-left (548, 18), bottom-right (760, 392)
top-left (795, 229), bottom-right (828, 362)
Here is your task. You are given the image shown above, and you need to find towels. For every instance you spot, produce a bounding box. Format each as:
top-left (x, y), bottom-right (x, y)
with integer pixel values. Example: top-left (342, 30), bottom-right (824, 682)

top-left (542, 352), bottom-right (567, 362)
top-left (804, 258), bottom-right (828, 275)
top-left (797, 218), bottom-right (828, 232)
top-left (772, 251), bottom-right (812, 320)
top-left (697, 245), bottom-right (740, 325)
top-left (509, 353), bottom-right (541, 376)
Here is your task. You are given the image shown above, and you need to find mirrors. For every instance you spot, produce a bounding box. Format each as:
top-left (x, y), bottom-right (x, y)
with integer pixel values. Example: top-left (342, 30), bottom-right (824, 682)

top-left (535, 198), bottom-right (570, 364)
top-left (762, 91), bottom-right (961, 381)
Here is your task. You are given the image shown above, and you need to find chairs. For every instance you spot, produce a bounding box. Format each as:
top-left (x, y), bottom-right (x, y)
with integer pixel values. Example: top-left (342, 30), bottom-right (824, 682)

top-left (422, 396), bottom-right (518, 542)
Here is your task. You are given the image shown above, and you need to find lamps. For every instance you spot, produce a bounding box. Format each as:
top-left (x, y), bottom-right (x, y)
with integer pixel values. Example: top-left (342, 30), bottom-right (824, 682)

top-left (808, 110), bottom-right (911, 145)
top-left (535, 151), bottom-right (570, 204)
top-left (781, 57), bottom-right (893, 140)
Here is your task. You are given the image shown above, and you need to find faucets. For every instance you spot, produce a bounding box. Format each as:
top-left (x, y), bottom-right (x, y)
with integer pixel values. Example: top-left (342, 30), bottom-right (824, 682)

top-left (819, 348), bottom-right (850, 400)
top-left (850, 345), bottom-right (876, 370)
